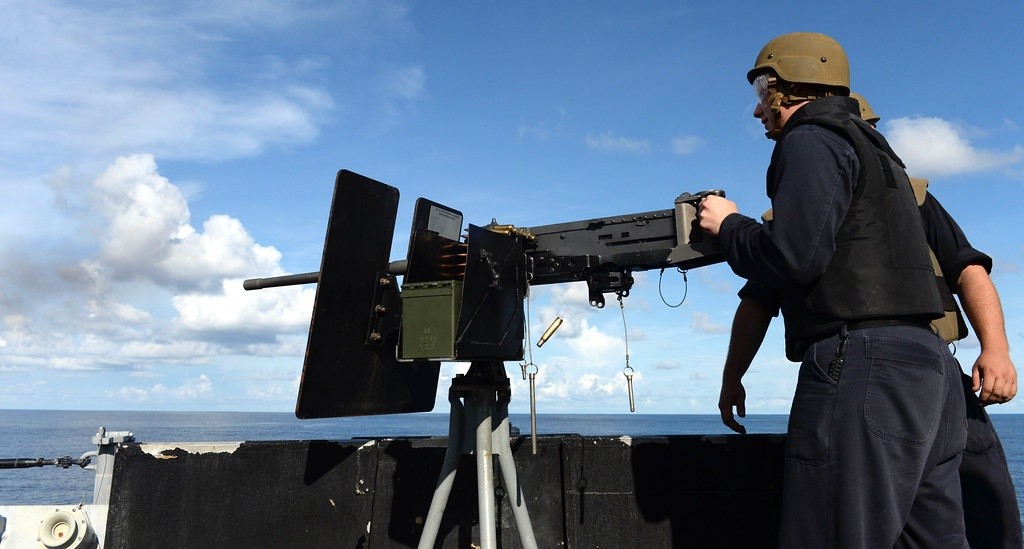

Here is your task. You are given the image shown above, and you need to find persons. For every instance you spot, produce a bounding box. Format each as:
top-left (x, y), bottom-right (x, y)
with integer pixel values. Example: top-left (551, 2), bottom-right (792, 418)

top-left (696, 31), bottom-right (967, 548)
top-left (718, 93), bottom-right (1023, 548)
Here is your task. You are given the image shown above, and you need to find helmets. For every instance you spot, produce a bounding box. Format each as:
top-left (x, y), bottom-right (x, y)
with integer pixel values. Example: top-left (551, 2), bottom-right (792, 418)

top-left (746, 32), bottom-right (850, 95)
top-left (849, 93), bottom-right (880, 121)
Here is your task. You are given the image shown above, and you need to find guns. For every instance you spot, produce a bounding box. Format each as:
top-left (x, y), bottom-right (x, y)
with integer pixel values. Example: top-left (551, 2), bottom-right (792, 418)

top-left (0, 454), bottom-right (92, 473)
top-left (246, 189), bottom-right (725, 360)
